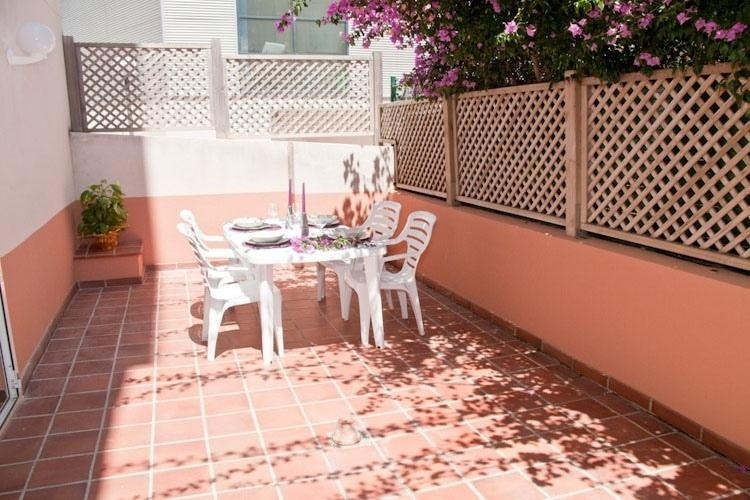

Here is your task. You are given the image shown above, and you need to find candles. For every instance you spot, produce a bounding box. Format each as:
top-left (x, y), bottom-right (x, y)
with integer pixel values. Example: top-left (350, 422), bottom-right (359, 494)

top-left (302, 182), bottom-right (305, 213)
top-left (289, 179), bottom-right (292, 205)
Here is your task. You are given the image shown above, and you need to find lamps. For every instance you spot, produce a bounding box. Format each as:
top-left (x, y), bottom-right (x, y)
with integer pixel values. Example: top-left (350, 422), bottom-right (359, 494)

top-left (7, 19), bottom-right (56, 65)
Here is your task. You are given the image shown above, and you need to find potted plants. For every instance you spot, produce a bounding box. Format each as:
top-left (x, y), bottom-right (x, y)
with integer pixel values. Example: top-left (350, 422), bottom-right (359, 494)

top-left (76, 176), bottom-right (130, 251)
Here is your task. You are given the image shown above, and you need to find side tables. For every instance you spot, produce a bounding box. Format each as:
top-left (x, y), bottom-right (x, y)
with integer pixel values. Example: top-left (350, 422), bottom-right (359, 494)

top-left (73, 238), bottom-right (145, 287)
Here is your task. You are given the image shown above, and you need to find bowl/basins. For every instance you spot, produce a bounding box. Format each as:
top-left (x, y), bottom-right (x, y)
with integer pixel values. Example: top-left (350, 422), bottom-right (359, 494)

top-left (335, 227), bottom-right (368, 238)
top-left (233, 217), bottom-right (265, 228)
top-left (309, 214), bottom-right (338, 224)
top-left (249, 231), bottom-right (285, 242)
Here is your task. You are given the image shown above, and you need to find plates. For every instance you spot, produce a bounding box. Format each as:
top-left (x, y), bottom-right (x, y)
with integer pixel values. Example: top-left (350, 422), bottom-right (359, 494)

top-left (245, 236), bottom-right (289, 246)
top-left (308, 220), bottom-right (340, 226)
top-left (232, 222), bottom-right (271, 230)
top-left (328, 231), bottom-right (368, 240)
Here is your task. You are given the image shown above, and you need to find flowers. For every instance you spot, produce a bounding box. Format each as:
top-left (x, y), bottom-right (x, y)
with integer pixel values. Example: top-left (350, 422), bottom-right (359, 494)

top-left (289, 234), bottom-right (363, 254)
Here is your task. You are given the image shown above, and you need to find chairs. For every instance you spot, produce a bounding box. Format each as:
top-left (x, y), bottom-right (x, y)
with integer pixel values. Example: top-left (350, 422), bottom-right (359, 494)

top-left (343, 210), bottom-right (437, 347)
top-left (176, 221), bottom-right (285, 361)
top-left (317, 200), bottom-right (402, 320)
top-left (180, 208), bottom-right (255, 283)
